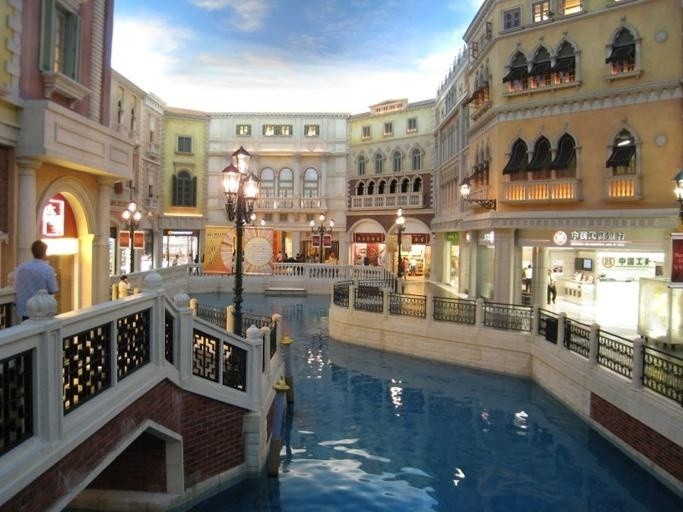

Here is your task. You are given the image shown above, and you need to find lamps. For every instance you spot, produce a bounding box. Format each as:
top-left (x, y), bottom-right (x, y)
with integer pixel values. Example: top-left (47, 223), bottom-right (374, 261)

top-left (459, 179), bottom-right (495, 210)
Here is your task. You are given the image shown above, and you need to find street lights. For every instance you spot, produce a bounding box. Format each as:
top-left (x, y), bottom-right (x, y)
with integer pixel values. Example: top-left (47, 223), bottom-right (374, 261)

top-left (220, 144), bottom-right (261, 365)
top-left (309, 211), bottom-right (335, 273)
top-left (395, 206), bottom-right (407, 278)
top-left (122, 200), bottom-right (142, 277)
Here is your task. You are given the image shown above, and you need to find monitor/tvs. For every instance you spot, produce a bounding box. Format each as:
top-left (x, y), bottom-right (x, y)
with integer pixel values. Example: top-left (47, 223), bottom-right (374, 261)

top-left (583, 259), bottom-right (592, 270)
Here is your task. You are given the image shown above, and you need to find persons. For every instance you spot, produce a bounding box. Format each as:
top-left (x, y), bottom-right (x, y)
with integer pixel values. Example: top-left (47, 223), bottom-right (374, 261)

top-left (172, 255), bottom-right (179, 266)
top-left (400, 256), bottom-right (408, 272)
top-left (15, 240), bottom-right (60, 321)
top-left (188, 254), bottom-right (198, 276)
top-left (118, 275), bottom-right (131, 298)
top-left (277, 248), bottom-right (338, 265)
top-left (547, 269), bottom-right (556, 304)
top-left (525, 265), bottom-right (532, 292)
